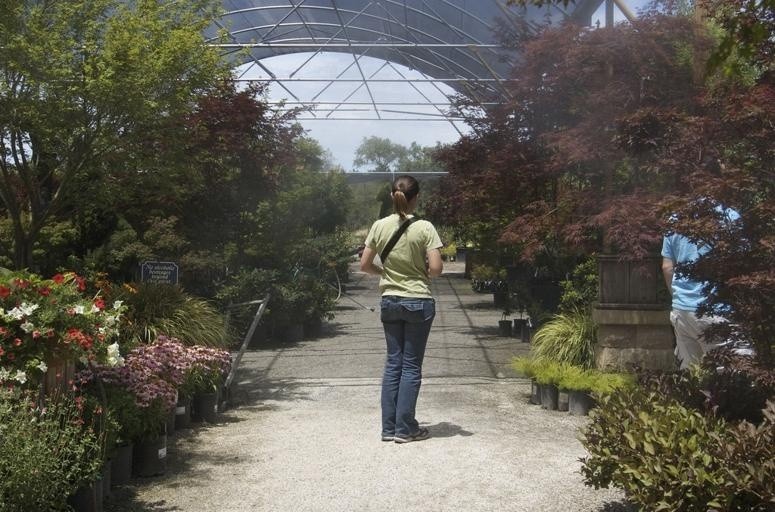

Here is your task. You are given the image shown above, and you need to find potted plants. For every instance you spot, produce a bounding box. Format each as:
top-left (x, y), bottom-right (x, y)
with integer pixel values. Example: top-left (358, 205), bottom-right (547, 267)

top-left (510, 357), bottom-right (601, 417)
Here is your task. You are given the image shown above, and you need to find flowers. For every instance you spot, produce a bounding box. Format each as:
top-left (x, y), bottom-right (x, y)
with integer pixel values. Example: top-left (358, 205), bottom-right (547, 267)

top-left (0, 272), bottom-right (233, 512)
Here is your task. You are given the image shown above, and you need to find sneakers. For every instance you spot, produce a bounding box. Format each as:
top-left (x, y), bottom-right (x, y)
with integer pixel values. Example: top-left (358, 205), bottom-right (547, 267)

top-left (381, 427), bottom-right (431, 444)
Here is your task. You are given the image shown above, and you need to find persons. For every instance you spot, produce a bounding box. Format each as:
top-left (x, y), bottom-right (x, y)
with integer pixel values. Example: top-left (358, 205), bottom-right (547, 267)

top-left (660, 158), bottom-right (757, 394)
top-left (357, 175), bottom-right (446, 444)
